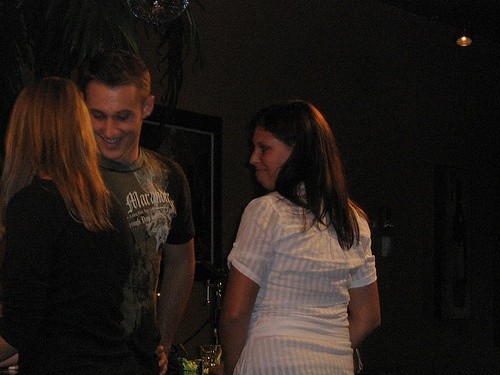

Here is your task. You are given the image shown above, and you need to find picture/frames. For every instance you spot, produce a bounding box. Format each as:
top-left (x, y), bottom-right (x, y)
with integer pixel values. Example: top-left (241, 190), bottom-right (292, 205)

top-left (138, 104), bottom-right (222, 282)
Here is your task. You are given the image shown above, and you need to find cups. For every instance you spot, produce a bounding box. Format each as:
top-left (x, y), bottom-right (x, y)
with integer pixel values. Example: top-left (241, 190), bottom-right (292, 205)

top-left (200, 345), bottom-right (222, 375)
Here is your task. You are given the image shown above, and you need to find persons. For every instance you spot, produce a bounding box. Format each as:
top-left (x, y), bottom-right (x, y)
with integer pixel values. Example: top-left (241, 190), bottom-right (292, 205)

top-left (0, 76), bottom-right (135, 375)
top-left (76, 48), bottom-right (195, 375)
top-left (219, 99), bottom-right (381, 375)
